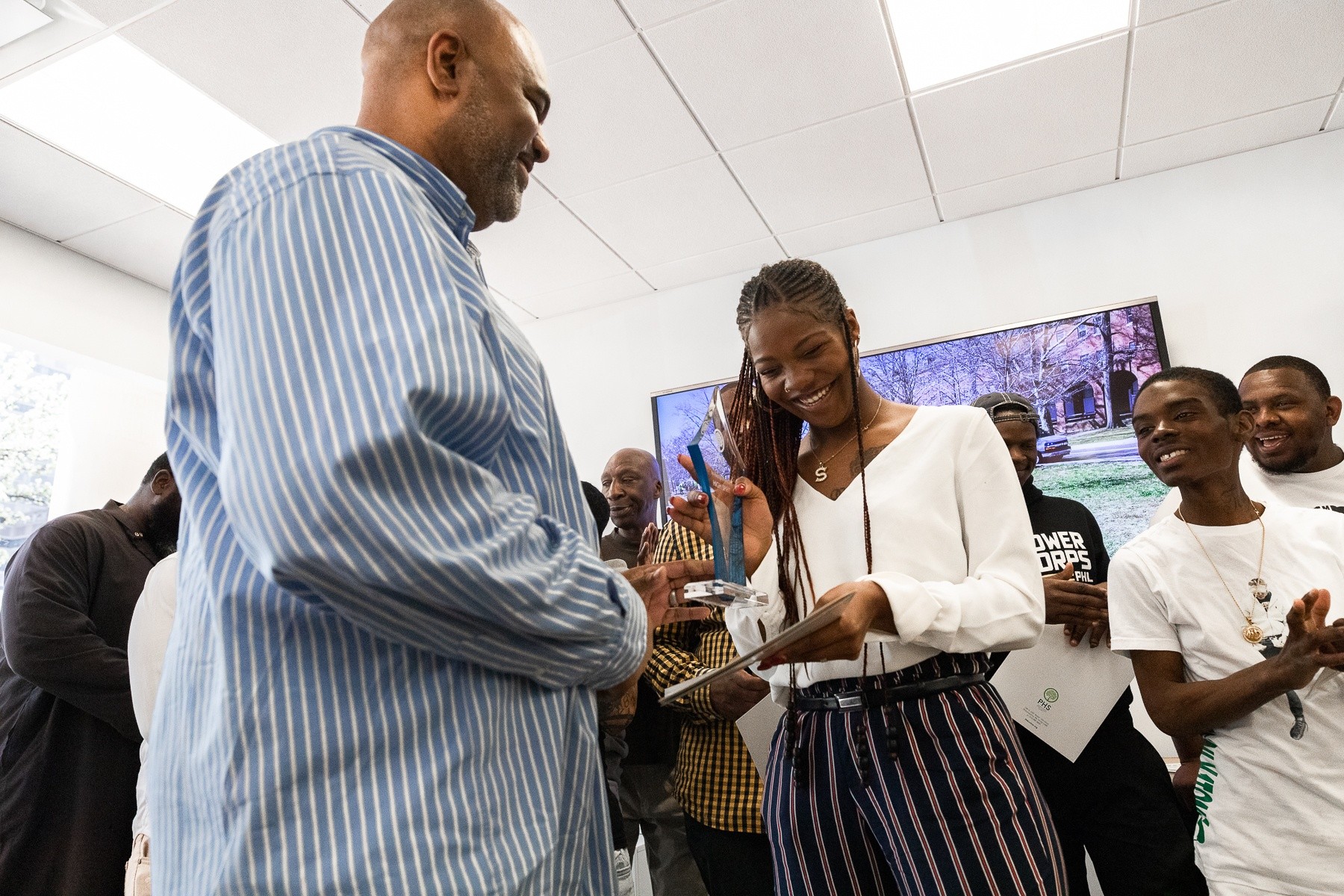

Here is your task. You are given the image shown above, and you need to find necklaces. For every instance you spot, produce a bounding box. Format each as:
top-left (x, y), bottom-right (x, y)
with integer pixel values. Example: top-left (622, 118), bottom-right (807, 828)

top-left (807, 388), bottom-right (884, 485)
top-left (1177, 495), bottom-right (1267, 648)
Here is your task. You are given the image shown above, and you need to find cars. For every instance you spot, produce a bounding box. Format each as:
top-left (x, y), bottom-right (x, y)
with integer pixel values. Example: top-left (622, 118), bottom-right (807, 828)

top-left (1036, 427), bottom-right (1071, 464)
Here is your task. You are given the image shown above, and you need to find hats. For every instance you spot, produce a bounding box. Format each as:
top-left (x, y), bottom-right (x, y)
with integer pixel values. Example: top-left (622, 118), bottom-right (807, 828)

top-left (971, 393), bottom-right (1040, 439)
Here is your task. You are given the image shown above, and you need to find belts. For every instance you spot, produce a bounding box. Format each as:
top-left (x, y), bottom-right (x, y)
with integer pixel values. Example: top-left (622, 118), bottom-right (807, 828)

top-left (793, 672), bottom-right (984, 712)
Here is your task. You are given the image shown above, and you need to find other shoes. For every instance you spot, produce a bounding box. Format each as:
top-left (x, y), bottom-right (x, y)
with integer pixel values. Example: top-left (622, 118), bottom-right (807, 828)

top-left (613, 850), bottom-right (635, 891)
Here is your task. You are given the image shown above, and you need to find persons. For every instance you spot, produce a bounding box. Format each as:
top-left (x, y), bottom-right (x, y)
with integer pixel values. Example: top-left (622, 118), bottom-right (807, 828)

top-left (969, 392), bottom-right (1188, 896)
top-left (664, 258), bottom-right (1070, 896)
top-left (147, 0), bottom-right (672, 896)
top-left (1107, 366), bottom-right (1344, 895)
top-left (0, 449), bottom-right (182, 896)
top-left (596, 448), bottom-right (707, 896)
top-left (643, 381), bottom-right (776, 896)
top-left (1155, 354), bottom-right (1344, 522)
